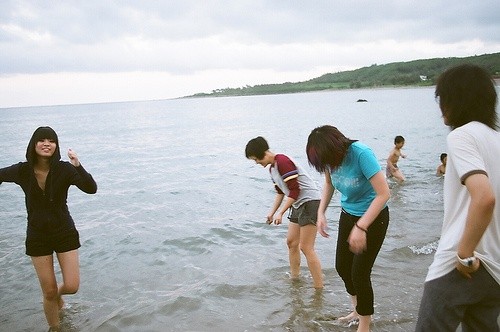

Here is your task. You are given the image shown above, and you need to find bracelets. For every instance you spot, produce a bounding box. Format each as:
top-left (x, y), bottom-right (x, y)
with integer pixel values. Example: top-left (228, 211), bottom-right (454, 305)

top-left (356, 223), bottom-right (367, 233)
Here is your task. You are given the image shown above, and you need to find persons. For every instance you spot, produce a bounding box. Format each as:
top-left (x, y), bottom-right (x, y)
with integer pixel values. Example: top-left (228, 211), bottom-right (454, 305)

top-left (245, 136), bottom-right (323, 288)
top-left (0, 126), bottom-right (97, 327)
top-left (436, 153), bottom-right (448, 177)
top-left (305, 125), bottom-right (390, 332)
top-left (414, 64), bottom-right (500, 332)
top-left (385, 135), bottom-right (407, 184)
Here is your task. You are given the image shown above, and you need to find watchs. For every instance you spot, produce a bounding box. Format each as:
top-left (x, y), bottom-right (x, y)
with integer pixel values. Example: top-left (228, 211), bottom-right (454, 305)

top-left (457, 253), bottom-right (476, 268)
